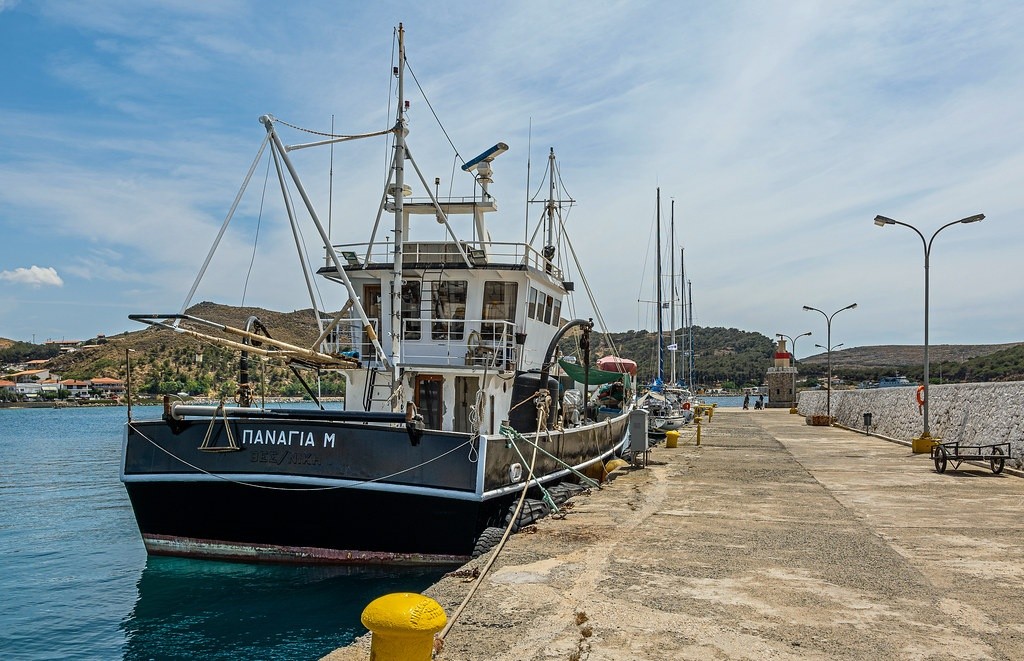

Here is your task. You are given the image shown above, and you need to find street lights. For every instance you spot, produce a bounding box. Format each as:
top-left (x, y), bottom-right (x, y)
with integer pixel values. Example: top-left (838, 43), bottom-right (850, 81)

top-left (775, 332), bottom-right (812, 416)
top-left (802, 303), bottom-right (858, 427)
top-left (874, 212), bottom-right (987, 454)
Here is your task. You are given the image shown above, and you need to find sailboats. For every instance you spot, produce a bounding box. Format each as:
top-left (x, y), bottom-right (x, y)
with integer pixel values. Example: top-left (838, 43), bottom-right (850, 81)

top-left (635, 184), bottom-right (707, 434)
top-left (119, 21), bottom-right (653, 566)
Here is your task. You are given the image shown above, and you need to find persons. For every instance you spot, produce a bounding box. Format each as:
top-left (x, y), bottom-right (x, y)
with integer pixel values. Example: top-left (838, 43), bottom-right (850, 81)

top-left (755, 395), bottom-right (764, 409)
top-left (743, 392), bottom-right (750, 410)
